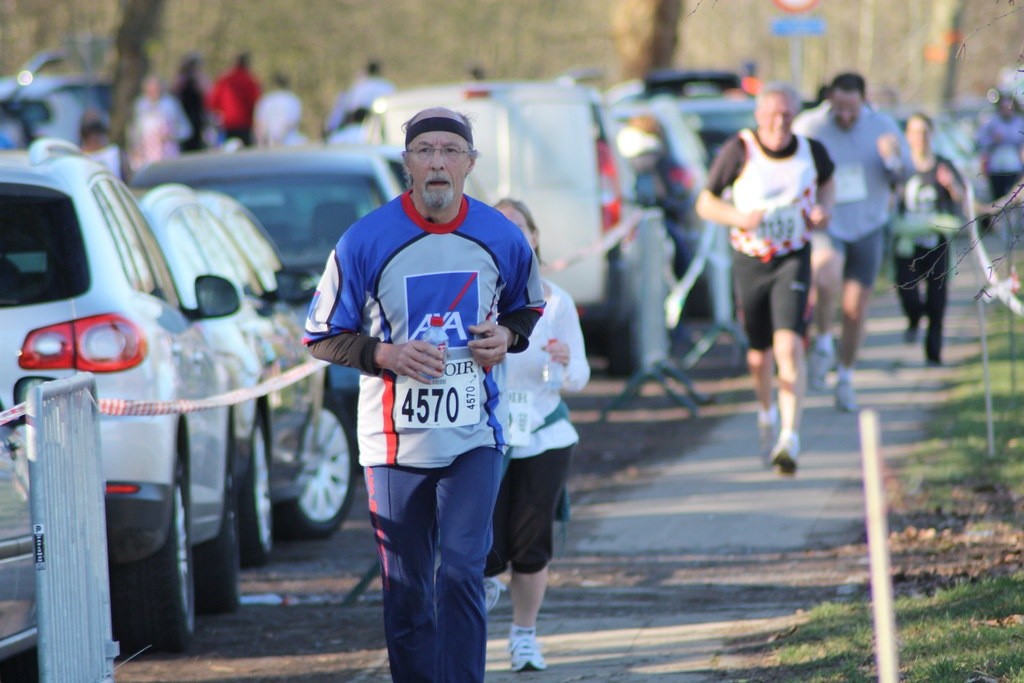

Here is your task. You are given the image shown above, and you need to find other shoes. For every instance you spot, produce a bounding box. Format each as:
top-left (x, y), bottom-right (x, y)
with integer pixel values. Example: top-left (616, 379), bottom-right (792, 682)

top-left (834, 379), bottom-right (860, 412)
top-left (769, 436), bottom-right (798, 475)
top-left (907, 316), bottom-right (923, 342)
top-left (757, 407), bottom-right (779, 442)
top-left (811, 342), bottom-right (835, 389)
top-left (926, 341), bottom-right (942, 368)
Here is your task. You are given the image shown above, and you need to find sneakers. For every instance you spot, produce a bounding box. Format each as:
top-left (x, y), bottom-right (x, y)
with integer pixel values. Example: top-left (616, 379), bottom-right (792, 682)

top-left (483, 575), bottom-right (500, 612)
top-left (508, 627), bottom-right (546, 671)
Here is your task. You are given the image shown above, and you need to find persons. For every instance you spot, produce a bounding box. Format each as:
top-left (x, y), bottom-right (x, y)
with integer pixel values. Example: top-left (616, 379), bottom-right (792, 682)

top-left (338, 58), bottom-right (397, 121)
top-left (884, 112), bottom-right (968, 366)
top-left (303, 107), bottom-right (546, 683)
top-left (470, 66), bottom-right (487, 82)
top-left (76, 110), bottom-right (121, 184)
top-left (788, 74), bottom-right (916, 411)
top-left (696, 82), bottom-right (835, 475)
top-left (740, 60), bottom-right (765, 96)
top-left (975, 93), bottom-right (1023, 216)
top-left (327, 108), bottom-right (370, 146)
top-left (481, 198), bottom-right (590, 671)
top-left (127, 49), bottom-right (308, 172)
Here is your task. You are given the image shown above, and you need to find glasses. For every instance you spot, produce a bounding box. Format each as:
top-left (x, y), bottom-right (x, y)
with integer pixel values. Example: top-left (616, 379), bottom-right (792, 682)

top-left (406, 144), bottom-right (472, 162)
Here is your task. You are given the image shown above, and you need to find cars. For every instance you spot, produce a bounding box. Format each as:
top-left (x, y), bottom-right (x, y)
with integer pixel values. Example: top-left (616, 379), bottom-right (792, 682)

top-left (0, 439), bottom-right (38, 660)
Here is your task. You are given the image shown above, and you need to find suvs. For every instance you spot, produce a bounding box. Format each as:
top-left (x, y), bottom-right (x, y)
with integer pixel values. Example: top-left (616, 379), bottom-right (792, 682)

top-left (0, 69), bottom-right (807, 653)
